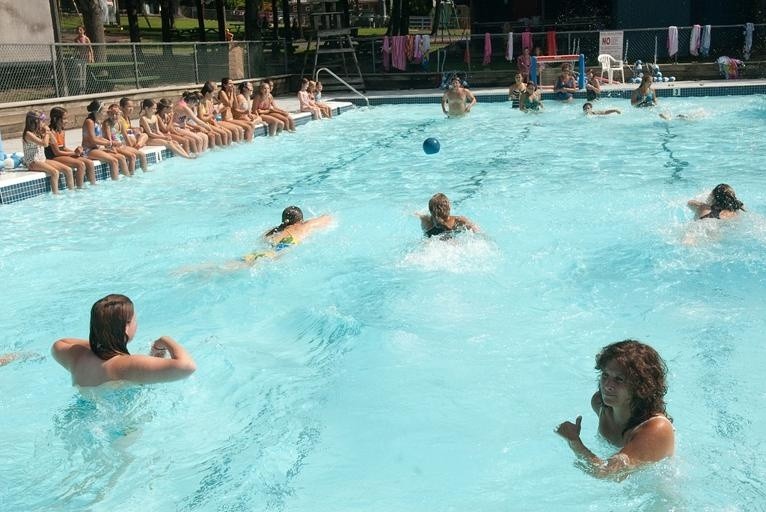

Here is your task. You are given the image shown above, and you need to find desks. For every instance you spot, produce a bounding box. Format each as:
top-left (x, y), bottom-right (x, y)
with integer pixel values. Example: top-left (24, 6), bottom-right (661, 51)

top-left (85, 61), bottom-right (146, 92)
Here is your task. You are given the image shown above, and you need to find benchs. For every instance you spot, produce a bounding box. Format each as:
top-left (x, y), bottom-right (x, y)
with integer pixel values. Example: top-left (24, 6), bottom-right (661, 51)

top-left (97, 75), bottom-right (160, 84)
top-left (177, 23), bottom-right (245, 40)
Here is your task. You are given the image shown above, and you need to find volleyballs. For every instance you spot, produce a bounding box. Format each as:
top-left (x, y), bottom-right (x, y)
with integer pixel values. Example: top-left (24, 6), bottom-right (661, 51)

top-left (628, 64), bottom-right (675, 83)
top-left (424, 137), bottom-right (439, 154)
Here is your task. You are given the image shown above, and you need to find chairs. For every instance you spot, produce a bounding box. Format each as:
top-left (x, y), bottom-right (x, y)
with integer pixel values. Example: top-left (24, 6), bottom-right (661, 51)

top-left (597, 54), bottom-right (625, 84)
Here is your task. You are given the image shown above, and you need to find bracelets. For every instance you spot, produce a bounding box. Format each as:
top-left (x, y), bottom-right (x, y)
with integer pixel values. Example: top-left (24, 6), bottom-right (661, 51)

top-left (153, 347), bottom-right (166, 351)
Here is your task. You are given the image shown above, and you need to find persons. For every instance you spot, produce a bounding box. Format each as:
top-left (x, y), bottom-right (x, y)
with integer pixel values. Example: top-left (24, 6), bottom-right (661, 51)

top-left (74, 25), bottom-right (94, 64)
top-left (226, 29), bottom-right (234, 41)
top-left (687, 183), bottom-right (748, 219)
top-left (420, 193), bottom-right (480, 241)
top-left (553, 339), bottom-right (676, 484)
top-left (50, 294), bottom-right (198, 388)
top-left (0, 350), bottom-right (43, 367)
top-left (174, 206), bottom-right (331, 276)
top-left (441, 46), bottom-right (710, 121)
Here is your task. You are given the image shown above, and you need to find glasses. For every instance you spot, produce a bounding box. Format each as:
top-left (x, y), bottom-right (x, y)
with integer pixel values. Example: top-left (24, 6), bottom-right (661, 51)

top-left (94, 101), bottom-right (105, 115)
top-left (26, 111), bottom-right (48, 120)
